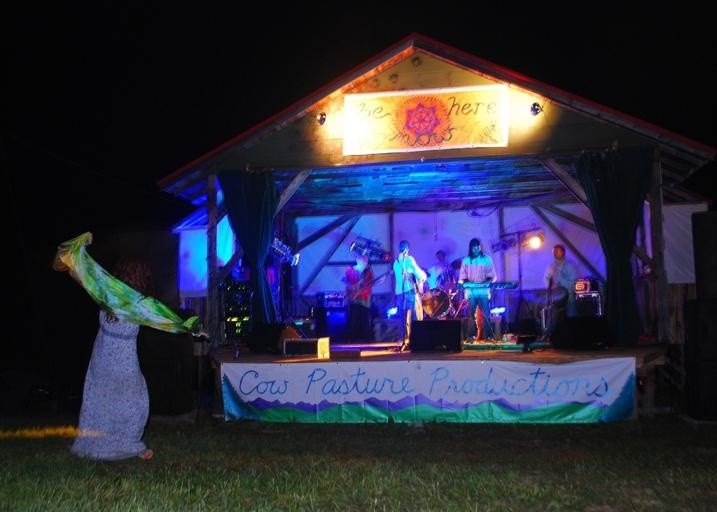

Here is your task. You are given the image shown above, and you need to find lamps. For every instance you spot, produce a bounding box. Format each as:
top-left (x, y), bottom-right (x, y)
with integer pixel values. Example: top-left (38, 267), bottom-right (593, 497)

top-left (531, 97), bottom-right (547, 115)
top-left (313, 112), bottom-right (326, 126)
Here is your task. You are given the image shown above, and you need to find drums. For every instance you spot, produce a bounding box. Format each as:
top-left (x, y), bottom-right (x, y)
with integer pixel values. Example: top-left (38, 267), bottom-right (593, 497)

top-left (423, 288), bottom-right (451, 317)
top-left (437, 271), bottom-right (458, 295)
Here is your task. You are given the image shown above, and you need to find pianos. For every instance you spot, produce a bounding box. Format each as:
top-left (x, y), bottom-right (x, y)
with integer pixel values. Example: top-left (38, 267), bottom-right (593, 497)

top-left (462, 281), bottom-right (519, 290)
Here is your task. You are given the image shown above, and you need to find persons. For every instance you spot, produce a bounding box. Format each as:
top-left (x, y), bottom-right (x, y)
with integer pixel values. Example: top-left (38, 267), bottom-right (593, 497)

top-left (458, 238), bottom-right (498, 343)
top-left (50, 231), bottom-right (211, 462)
top-left (538, 245), bottom-right (581, 340)
top-left (392, 239), bottom-right (427, 343)
top-left (424, 249), bottom-right (458, 319)
top-left (342, 255), bottom-right (392, 344)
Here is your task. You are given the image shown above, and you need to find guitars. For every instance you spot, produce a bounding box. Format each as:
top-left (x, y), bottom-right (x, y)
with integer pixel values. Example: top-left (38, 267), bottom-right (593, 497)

top-left (347, 271), bottom-right (391, 301)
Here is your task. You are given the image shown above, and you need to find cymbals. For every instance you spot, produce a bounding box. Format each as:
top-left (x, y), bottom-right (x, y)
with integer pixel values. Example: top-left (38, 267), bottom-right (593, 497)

top-left (451, 256), bottom-right (466, 269)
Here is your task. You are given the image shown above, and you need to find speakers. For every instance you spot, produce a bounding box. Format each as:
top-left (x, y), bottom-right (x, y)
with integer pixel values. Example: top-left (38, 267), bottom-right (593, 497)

top-left (691, 212), bottom-right (717, 300)
top-left (284, 339), bottom-right (318, 354)
top-left (409, 320), bottom-right (463, 353)
top-left (684, 301), bottom-right (717, 420)
top-left (314, 307), bottom-right (351, 343)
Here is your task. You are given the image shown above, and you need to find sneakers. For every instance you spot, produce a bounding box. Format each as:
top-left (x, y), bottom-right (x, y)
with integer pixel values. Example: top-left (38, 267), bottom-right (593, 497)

top-left (137, 448), bottom-right (153, 461)
